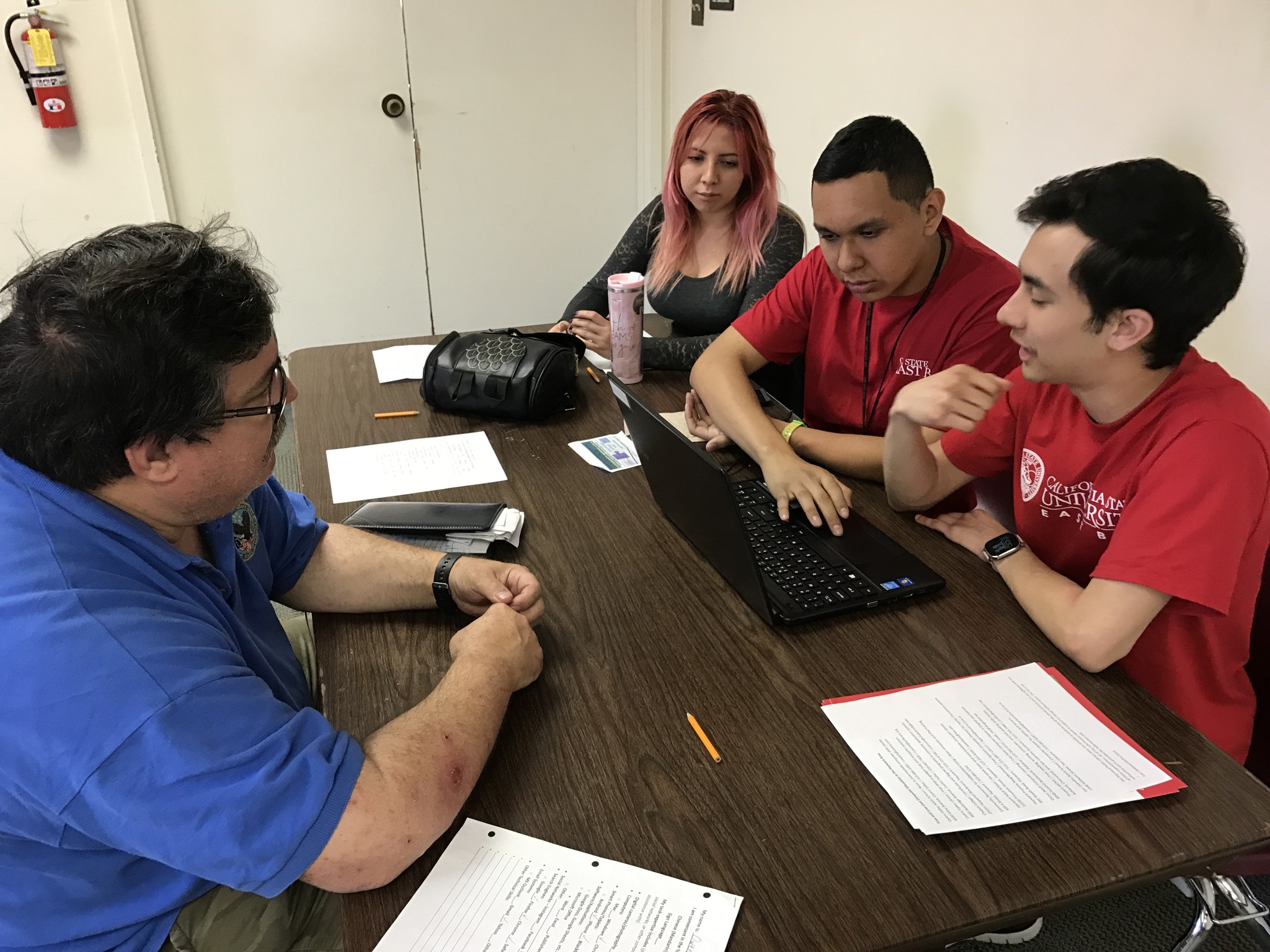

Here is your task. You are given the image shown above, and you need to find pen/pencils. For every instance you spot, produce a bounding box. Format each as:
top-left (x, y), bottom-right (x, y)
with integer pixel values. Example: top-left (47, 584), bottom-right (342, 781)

top-left (685, 712), bottom-right (721, 763)
top-left (374, 411), bottom-right (420, 419)
top-left (587, 367), bottom-right (600, 383)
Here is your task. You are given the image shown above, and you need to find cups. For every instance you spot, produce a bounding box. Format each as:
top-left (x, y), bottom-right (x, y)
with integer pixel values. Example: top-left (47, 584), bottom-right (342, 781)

top-left (607, 271), bottom-right (645, 384)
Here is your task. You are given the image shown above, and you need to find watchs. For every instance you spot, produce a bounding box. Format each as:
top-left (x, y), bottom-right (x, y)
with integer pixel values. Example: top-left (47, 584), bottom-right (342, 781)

top-left (981, 532), bottom-right (1031, 572)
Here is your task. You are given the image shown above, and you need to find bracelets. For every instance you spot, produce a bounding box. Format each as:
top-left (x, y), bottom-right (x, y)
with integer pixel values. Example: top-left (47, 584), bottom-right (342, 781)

top-left (431, 552), bottom-right (466, 612)
top-left (780, 420), bottom-right (807, 443)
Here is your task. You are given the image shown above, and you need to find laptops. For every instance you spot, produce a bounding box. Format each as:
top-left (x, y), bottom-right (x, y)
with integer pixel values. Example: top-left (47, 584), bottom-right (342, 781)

top-left (602, 371), bottom-right (946, 624)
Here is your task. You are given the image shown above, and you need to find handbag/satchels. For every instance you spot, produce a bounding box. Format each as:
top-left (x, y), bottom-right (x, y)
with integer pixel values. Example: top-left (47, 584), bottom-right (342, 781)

top-left (418, 326), bottom-right (587, 423)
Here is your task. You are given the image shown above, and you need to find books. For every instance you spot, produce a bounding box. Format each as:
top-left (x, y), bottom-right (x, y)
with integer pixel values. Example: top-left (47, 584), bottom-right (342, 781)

top-left (337, 495), bottom-right (507, 562)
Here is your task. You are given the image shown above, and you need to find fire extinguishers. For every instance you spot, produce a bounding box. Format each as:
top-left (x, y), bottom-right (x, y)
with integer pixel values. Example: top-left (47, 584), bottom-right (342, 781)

top-left (4, 0), bottom-right (80, 128)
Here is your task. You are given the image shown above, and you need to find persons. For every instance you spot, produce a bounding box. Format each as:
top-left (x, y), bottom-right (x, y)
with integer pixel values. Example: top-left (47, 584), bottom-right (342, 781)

top-left (883, 159), bottom-right (1270, 946)
top-left (683, 116), bottom-right (1018, 539)
top-left (547, 91), bottom-right (806, 377)
top-left (0, 215), bottom-right (540, 952)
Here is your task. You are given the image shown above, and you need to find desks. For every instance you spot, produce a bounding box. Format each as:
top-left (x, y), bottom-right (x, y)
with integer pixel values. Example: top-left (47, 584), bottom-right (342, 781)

top-left (289, 313), bottom-right (1270, 952)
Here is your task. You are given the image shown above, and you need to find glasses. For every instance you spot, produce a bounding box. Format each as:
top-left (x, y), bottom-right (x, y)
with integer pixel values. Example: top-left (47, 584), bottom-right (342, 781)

top-left (191, 362), bottom-right (289, 425)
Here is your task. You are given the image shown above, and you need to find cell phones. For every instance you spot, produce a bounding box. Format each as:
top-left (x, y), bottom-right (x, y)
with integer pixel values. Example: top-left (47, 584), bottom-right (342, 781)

top-left (753, 387), bottom-right (773, 407)
